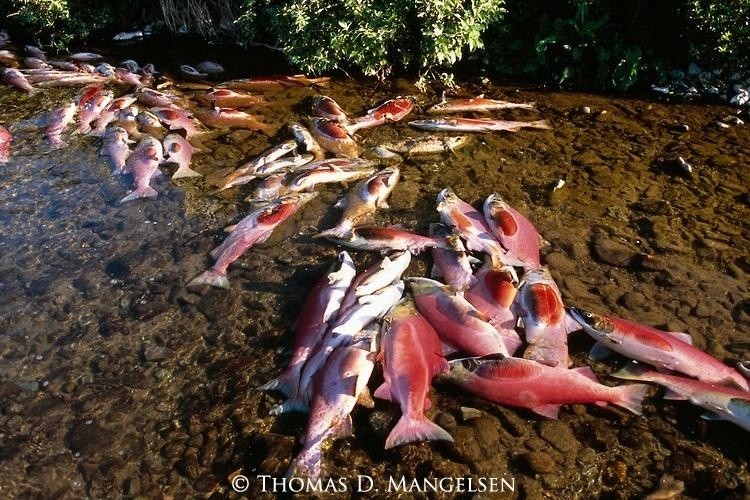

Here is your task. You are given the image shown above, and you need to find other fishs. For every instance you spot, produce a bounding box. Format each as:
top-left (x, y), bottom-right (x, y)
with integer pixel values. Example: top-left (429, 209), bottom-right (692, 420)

top-left (0, 45), bottom-right (750, 500)
